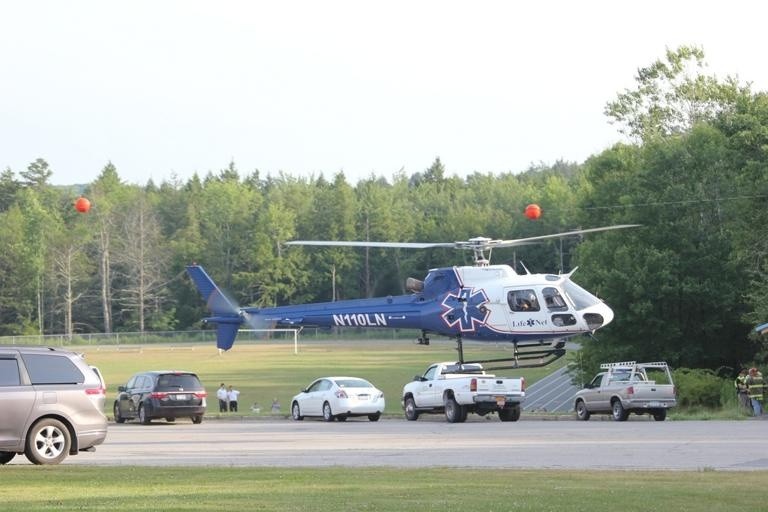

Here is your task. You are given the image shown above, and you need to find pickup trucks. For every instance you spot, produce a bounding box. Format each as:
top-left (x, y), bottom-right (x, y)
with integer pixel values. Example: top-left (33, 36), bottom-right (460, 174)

top-left (399, 361), bottom-right (525, 421)
top-left (573, 360), bottom-right (676, 421)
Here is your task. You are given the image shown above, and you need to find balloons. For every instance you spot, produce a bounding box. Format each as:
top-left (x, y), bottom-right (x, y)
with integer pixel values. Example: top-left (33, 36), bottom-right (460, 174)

top-left (75, 198), bottom-right (90, 213)
top-left (524, 204), bottom-right (541, 219)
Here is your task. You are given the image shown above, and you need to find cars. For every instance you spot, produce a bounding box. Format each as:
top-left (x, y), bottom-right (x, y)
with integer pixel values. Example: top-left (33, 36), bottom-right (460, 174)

top-left (290, 376), bottom-right (385, 421)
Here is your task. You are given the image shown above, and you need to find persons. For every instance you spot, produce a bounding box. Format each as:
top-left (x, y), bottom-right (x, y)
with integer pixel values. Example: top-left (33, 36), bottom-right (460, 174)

top-left (248, 401), bottom-right (264, 413)
top-left (217, 383), bottom-right (227, 412)
top-left (226, 385), bottom-right (240, 413)
top-left (269, 397), bottom-right (280, 413)
top-left (734, 368), bottom-right (763, 417)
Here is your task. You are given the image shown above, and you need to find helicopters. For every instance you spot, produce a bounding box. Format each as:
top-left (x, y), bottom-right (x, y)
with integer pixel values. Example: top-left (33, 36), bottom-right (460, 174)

top-left (185, 224), bottom-right (644, 375)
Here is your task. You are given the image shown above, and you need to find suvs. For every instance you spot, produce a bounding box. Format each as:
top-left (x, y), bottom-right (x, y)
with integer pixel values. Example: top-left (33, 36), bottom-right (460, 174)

top-left (1, 344), bottom-right (108, 465)
top-left (113, 370), bottom-right (208, 424)
